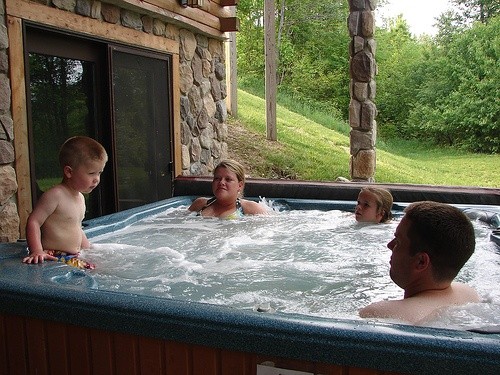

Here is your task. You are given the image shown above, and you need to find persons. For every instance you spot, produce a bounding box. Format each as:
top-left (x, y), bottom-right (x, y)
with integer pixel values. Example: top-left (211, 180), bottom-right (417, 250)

top-left (358, 201), bottom-right (482, 324)
top-left (353, 187), bottom-right (393, 224)
top-left (188, 159), bottom-right (264, 219)
top-left (22, 136), bottom-right (108, 269)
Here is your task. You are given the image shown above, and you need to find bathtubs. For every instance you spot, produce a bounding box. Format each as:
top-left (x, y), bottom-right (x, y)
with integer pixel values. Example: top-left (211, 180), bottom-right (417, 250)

top-left (1, 196), bottom-right (499, 373)
